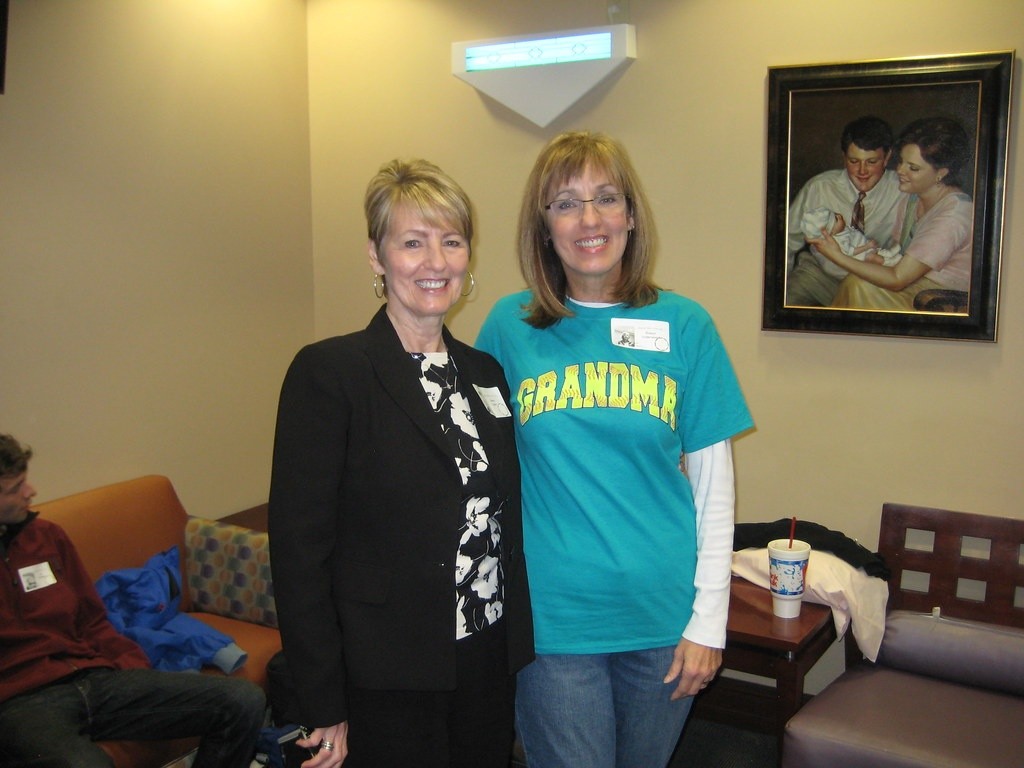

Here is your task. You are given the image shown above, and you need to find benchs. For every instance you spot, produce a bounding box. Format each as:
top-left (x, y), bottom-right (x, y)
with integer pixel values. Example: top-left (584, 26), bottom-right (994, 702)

top-left (778, 501), bottom-right (1024, 768)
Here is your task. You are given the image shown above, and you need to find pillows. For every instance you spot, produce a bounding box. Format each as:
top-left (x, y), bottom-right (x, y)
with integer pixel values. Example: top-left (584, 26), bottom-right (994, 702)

top-left (878, 609), bottom-right (1024, 695)
top-left (183, 515), bottom-right (282, 629)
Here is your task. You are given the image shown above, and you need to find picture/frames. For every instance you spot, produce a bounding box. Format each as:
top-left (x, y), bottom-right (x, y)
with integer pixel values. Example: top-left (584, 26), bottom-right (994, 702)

top-left (760, 49), bottom-right (1016, 343)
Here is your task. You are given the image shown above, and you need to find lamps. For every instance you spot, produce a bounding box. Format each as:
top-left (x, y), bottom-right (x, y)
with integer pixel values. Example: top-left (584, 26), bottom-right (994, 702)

top-left (452, 23), bottom-right (637, 125)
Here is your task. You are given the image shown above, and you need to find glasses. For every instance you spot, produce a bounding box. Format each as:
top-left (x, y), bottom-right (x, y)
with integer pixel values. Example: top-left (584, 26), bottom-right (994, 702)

top-left (545, 193), bottom-right (626, 218)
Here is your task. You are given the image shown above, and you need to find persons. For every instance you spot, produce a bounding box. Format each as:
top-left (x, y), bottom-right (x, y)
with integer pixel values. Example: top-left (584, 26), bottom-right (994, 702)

top-left (471, 131), bottom-right (755, 768)
top-left (266, 159), bottom-right (536, 768)
top-left (0, 434), bottom-right (266, 768)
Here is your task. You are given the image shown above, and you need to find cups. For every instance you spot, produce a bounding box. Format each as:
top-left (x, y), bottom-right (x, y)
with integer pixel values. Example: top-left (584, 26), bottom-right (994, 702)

top-left (768, 539), bottom-right (811, 619)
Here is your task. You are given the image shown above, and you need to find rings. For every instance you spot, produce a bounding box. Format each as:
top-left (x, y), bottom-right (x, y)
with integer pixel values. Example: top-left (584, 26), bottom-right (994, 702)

top-left (702, 681), bottom-right (709, 685)
top-left (321, 741), bottom-right (334, 751)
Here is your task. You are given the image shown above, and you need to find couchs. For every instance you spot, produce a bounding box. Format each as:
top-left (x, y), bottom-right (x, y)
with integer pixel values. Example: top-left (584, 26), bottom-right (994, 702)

top-left (1, 476), bottom-right (283, 767)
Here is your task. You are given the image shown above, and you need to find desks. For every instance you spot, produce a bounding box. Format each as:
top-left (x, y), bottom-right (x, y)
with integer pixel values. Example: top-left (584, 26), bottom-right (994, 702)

top-left (688, 576), bottom-right (866, 767)
